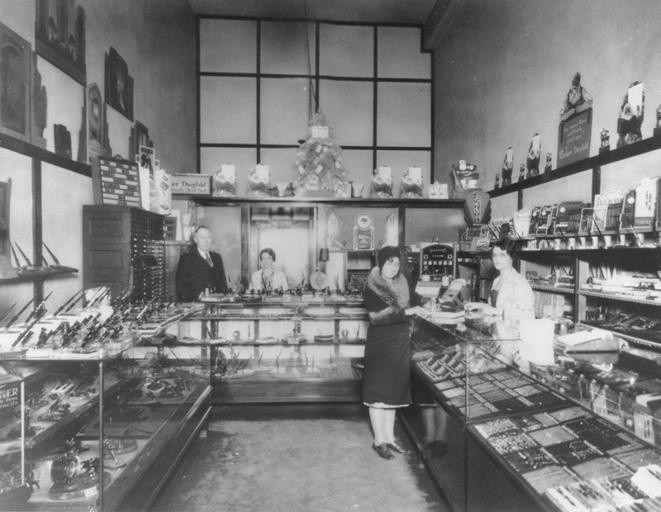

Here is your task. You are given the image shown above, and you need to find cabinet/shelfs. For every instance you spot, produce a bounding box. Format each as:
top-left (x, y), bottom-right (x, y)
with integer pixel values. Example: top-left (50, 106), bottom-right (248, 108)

top-left (179, 117), bottom-right (661, 442)
top-left (0, 288), bottom-right (368, 512)
top-left (74, 202), bottom-right (178, 315)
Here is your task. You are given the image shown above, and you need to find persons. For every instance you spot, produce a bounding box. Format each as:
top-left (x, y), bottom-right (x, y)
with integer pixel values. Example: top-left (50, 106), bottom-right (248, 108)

top-left (559, 70), bottom-right (593, 113)
top-left (174, 224), bottom-right (227, 304)
top-left (462, 239), bottom-right (536, 324)
top-left (246, 247), bottom-right (289, 294)
top-left (362, 246), bottom-right (435, 461)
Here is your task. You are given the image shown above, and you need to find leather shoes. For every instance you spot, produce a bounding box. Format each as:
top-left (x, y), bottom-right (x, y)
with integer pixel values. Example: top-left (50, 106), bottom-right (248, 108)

top-left (373, 439), bottom-right (405, 459)
